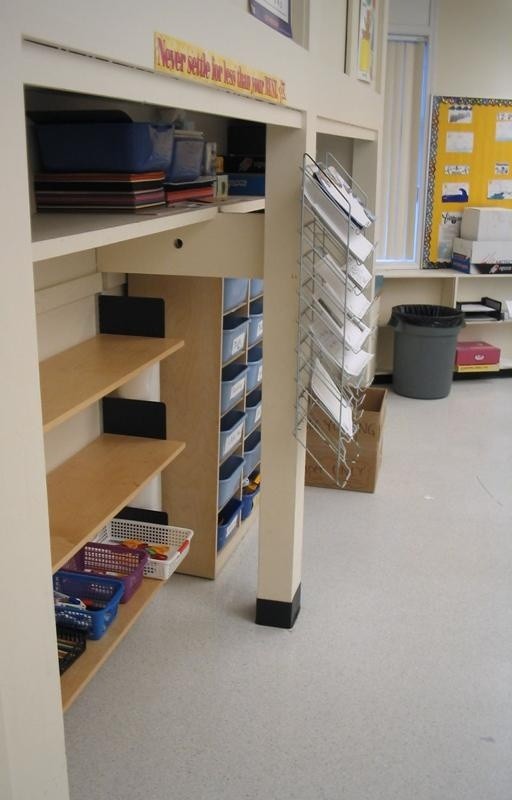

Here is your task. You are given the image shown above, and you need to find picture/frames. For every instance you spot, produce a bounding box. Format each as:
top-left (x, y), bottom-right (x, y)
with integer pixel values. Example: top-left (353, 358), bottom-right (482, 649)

top-left (345, 1), bottom-right (378, 83)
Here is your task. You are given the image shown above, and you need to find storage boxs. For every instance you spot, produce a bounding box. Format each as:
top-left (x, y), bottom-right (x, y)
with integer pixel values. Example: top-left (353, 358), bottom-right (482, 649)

top-left (305, 388), bottom-right (388, 493)
top-left (27, 120), bottom-right (175, 174)
top-left (166, 139), bottom-right (205, 182)
top-left (460, 206), bottom-right (512, 241)
top-left (452, 236), bottom-right (511, 274)
top-left (218, 279), bottom-right (264, 552)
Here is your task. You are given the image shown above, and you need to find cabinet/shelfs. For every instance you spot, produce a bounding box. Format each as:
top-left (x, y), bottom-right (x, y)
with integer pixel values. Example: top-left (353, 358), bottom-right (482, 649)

top-left (373, 268), bottom-right (511, 375)
top-left (124, 273), bottom-right (265, 580)
top-left (35, 333), bottom-right (195, 715)
top-left (298, 150), bottom-right (371, 487)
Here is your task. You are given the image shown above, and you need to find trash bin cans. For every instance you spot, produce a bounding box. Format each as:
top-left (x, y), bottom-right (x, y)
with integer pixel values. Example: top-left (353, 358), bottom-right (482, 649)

top-left (387, 305), bottom-right (465, 400)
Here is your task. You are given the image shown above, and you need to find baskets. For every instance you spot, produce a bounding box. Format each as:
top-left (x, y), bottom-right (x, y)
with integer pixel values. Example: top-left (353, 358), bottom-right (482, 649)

top-left (63, 542), bottom-right (150, 604)
top-left (89, 518), bottom-right (194, 581)
top-left (56, 622), bottom-right (86, 676)
top-left (53, 568), bottom-right (127, 641)
